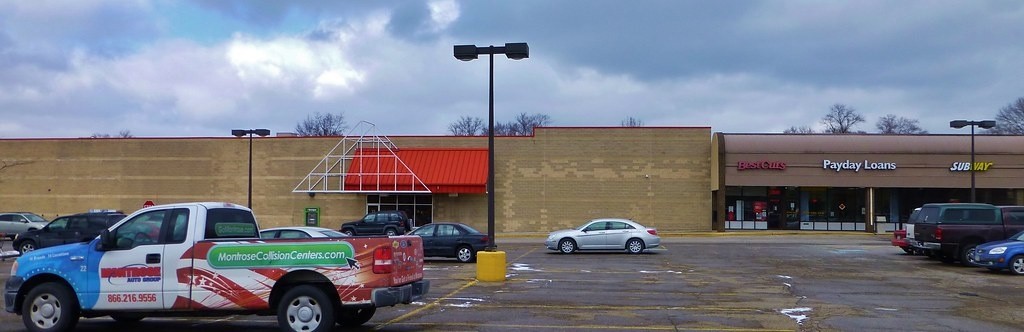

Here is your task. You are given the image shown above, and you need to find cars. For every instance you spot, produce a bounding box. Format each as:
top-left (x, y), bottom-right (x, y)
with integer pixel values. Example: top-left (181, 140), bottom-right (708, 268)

top-left (0, 211), bottom-right (50, 237)
top-left (966, 231), bottom-right (1024, 275)
top-left (11, 209), bottom-right (129, 255)
top-left (544, 218), bottom-right (661, 254)
top-left (408, 222), bottom-right (490, 262)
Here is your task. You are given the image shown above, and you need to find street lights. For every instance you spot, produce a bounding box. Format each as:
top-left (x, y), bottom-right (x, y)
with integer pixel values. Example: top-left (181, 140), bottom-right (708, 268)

top-left (231, 128), bottom-right (272, 209)
top-left (453, 41), bottom-right (530, 251)
top-left (949, 119), bottom-right (996, 203)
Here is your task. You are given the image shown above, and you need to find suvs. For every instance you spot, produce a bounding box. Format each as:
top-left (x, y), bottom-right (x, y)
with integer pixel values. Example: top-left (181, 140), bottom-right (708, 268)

top-left (341, 210), bottom-right (413, 236)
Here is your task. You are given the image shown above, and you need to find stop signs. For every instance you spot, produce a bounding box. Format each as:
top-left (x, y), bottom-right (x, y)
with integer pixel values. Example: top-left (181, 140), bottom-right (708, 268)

top-left (143, 201), bottom-right (156, 209)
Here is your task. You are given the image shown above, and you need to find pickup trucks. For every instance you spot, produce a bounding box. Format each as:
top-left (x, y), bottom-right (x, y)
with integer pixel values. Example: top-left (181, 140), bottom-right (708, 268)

top-left (913, 203), bottom-right (1024, 267)
top-left (4, 201), bottom-right (431, 332)
